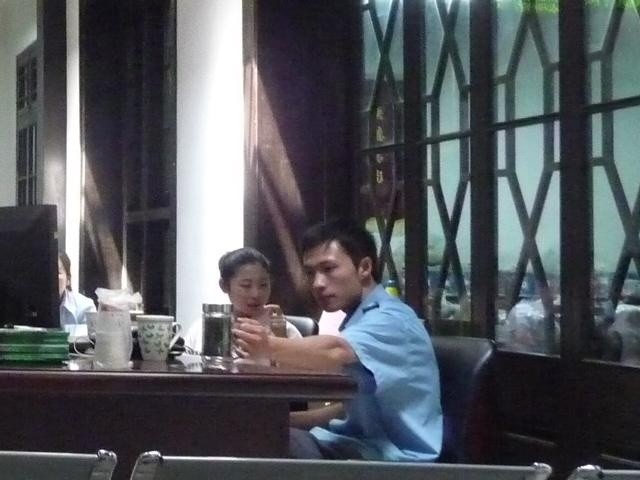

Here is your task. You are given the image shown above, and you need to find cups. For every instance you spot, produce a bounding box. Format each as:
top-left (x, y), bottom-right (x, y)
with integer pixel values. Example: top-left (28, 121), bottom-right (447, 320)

top-left (201, 302), bottom-right (250, 366)
top-left (73, 311), bottom-right (134, 370)
top-left (136, 314), bottom-right (183, 361)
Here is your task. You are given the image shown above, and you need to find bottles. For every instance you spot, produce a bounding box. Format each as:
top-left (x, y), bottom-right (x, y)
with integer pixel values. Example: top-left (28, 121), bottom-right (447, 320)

top-left (384, 279), bottom-right (399, 298)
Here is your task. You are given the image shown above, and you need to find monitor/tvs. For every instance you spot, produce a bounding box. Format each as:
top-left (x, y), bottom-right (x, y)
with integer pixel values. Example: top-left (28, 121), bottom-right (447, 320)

top-left (0, 203), bottom-right (61, 329)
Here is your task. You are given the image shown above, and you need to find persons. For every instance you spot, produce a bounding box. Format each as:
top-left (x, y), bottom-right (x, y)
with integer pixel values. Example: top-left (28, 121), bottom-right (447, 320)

top-left (231, 217), bottom-right (446, 462)
top-left (57, 248), bottom-right (97, 328)
top-left (397, 274), bottom-right (640, 368)
top-left (183, 246), bottom-right (303, 368)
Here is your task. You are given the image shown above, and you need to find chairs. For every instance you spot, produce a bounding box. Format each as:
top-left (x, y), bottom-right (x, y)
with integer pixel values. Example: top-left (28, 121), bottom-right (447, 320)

top-left (283, 312), bottom-right (319, 338)
top-left (427, 333), bottom-right (497, 459)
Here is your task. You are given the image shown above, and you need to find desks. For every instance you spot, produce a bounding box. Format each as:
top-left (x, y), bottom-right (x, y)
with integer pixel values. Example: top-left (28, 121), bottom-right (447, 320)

top-left (0, 352), bottom-right (361, 480)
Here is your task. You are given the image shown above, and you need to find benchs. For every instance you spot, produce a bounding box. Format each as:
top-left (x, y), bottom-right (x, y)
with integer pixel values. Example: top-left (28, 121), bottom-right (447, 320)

top-left (0, 450), bottom-right (640, 480)
